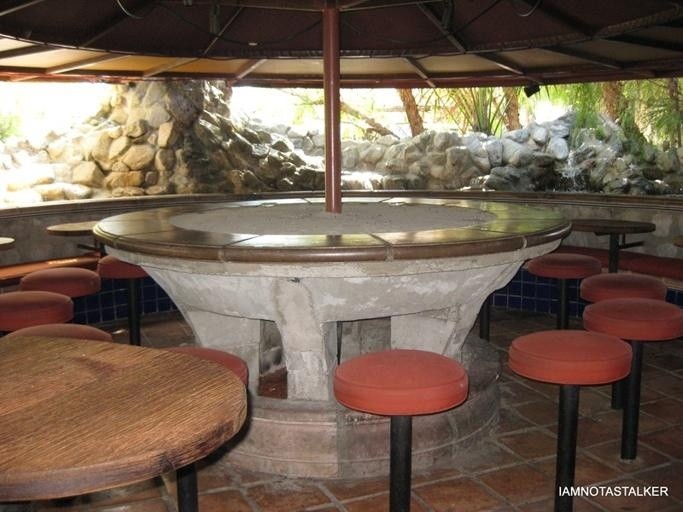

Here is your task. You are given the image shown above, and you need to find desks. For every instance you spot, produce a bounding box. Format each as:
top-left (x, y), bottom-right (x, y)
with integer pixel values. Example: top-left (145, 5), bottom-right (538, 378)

top-left (571, 219), bottom-right (656, 273)
top-left (45, 221), bottom-right (102, 256)
top-left (0, 238), bottom-right (15, 251)
top-left (91, 195), bottom-right (572, 478)
top-left (0, 336), bottom-right (248, 501)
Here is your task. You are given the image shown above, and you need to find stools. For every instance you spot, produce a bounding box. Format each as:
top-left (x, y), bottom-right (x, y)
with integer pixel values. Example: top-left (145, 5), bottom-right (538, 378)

top-left (507, 328), bottom-right (633, 510)
top-left (332, 347), bottom-right (469, 510)
top-left (97, 255), bottom-right (149, 345)
top-left (581, 298), bottom-right (682, 461)
top-left (0, 323), bottom-right (113, 343)
top-left (0, 291), bottom-right (75, 332)
top-left (19, 267), bottom-right (100, 300)
top-left (525, 253), bottom-right (600, 329)
top-left (581, 271), bottom-right (667, 411)
top-left (161, 345), bottom-right (248, 511)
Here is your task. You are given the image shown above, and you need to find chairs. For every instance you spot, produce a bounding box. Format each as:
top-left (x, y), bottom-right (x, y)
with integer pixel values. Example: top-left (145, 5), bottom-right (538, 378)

top-left (673, 234), bottom-right (683, 246)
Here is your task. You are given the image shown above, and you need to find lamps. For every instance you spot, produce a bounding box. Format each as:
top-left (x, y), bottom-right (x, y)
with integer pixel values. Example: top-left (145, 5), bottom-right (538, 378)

top-left (523, 83), bottom-right (541, 97)
